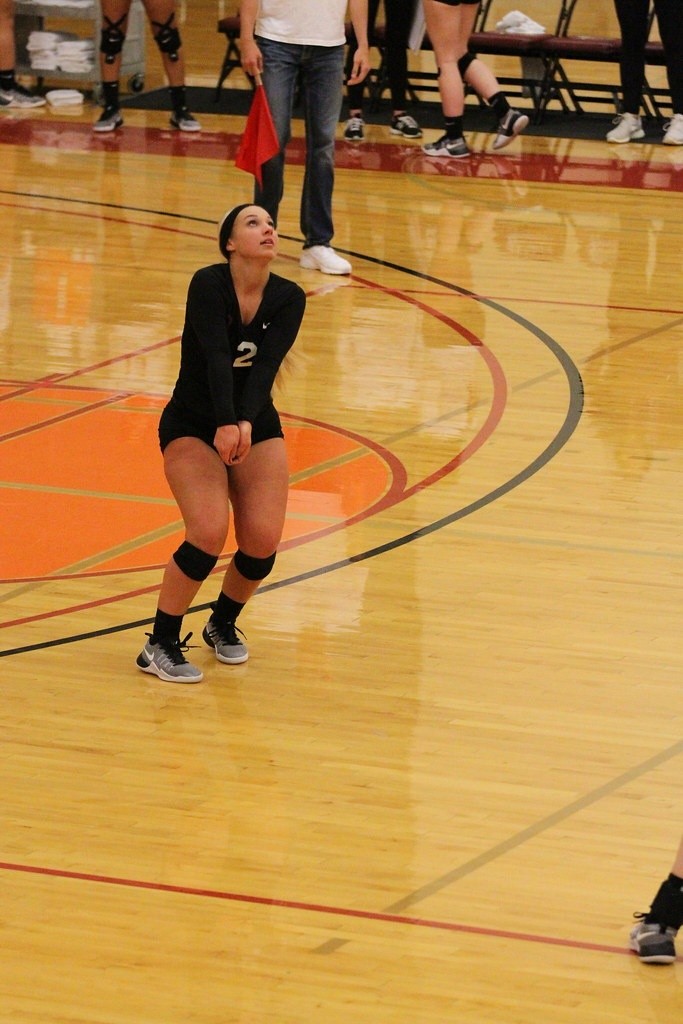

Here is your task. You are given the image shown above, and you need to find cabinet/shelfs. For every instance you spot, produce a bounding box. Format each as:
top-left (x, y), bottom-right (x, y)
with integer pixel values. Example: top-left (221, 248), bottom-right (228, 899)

top-left (12, 0), bottom-right (147, 106)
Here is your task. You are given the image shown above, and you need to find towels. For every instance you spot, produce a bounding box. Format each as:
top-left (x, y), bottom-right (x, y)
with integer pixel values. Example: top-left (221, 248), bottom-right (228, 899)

top-left (46, 88), bottom-right (84, 107)
top-left (56, 39), bottom-right (96, 73)
top-left (495, 7), bottom-right (551, 98)
top-left (26, 30), bottom-right (78, 69)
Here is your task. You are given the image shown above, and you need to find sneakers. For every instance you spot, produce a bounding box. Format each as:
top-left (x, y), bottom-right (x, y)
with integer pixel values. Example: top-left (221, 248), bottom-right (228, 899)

top-left (135, 631), bottom-right (203, 682)
top-left (661, 113), bottom-right (683, 144)
top-left (606, 112), bottom-right (645, 142)
top-left (426, 155), bottom-right (471, 180)
top-left (0, 87), bottom-right (47, 108)
top-left (389, 113), bottom-right (422, 139)
top-left (170, 112), bottom-right (201, 131)
top-left (300, 243), bottom-right (352, 274)
top-left (93, 106), bottom-right (124, 132)
top-left (420, 133), bottom-right (473, 158)
top-left (629, 912), bottom-right (676, 964)
top-left (302, 269), bottom-right (351, 297)
top-left (492, 109), bottom-right (531, 149)
top-left (343, 117), bottom-right (365, 141)
top-left (202, 602), bottom-right (249, 662)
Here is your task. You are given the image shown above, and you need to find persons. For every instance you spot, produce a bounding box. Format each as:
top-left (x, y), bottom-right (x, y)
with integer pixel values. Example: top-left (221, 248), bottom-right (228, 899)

top-left (605, 0), bottom-right (683, 146)
top-left (90, 0), bottom-right (202, 131)
top-left (629, 831), bottom-right (683, 963)
top-left (420, 0), bottom-right (528, 158)
top-left (344, 0), bottom-right (425, 140)
top-left (239, 0), bottom-right (371, 274)
top-left (136, 202), bottom-right (306, 684)
top-left (0, 0), bottom-right (45, 108)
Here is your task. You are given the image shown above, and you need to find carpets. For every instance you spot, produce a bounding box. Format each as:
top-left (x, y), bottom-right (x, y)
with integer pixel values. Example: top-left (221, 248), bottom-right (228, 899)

top-left (99, 85), bottom-right (673, 147)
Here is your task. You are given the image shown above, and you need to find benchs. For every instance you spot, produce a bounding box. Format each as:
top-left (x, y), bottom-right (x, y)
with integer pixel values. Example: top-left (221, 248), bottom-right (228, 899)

top-left (213, 16), bottom-right (669, 119)
